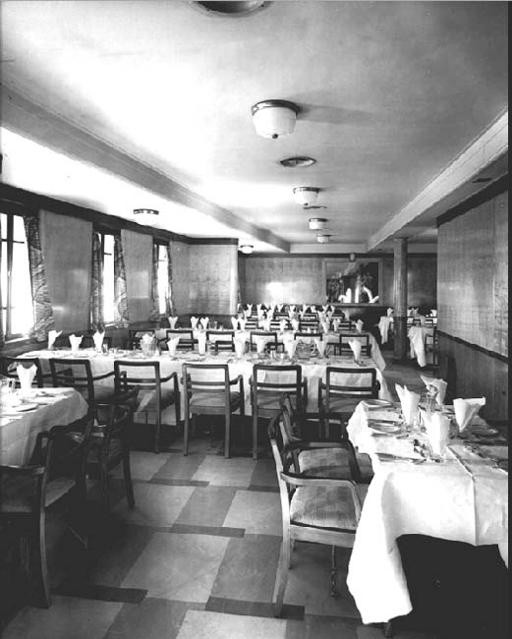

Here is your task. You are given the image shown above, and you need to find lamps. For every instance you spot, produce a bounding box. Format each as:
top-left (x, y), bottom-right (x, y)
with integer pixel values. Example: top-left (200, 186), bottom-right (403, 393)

top-left (290, 185), bottom-right (321, 209)
top-left (238, 242), bottom-right (256, 256)
top-left (131, 207), bottom-right (161, 228)
top-left (307, 217), bottom-right (328, 231)
top-left (317, 233), bottom-right (330, 243)
top-left (248, 96), bottom-right (301, 142)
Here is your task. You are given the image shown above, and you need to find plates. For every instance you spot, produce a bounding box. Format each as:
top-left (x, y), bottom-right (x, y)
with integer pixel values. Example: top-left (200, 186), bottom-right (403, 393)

top-left (471, 424), bottom-right (499, 437)
top-left (368, 422), bottom-right (398, 434)
top-left (0, 377), bottom-right (55, 416)
top-left (362, 398), bottom-right (391, 409)
top-left (496, 458), bottom-right (508, 475)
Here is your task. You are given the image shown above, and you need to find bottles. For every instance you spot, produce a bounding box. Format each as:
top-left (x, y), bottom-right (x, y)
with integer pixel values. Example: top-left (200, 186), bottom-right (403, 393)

top-left (294, 327), bottom-right (319, 335)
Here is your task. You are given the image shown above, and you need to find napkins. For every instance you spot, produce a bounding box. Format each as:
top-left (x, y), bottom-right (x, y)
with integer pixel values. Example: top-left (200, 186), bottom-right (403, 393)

top-left (395, 383), bottom-right (421, 425)
top-left (420, 374), bottom-right (448, 403)
top-left (453, 396), bottom-right (486, 434)
top-left (421, 409), bottom-right (450, 455)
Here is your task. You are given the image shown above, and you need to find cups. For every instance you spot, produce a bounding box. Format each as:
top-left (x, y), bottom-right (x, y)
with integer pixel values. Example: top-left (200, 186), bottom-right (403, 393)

top-left (209, 320), bottom-right (217, 329)
top-left (198, 324), bottom-right (202, 329)
top-left (47, 338), bottom-right (362, 367)
top-left (401, 391), bottom-right (470, 463)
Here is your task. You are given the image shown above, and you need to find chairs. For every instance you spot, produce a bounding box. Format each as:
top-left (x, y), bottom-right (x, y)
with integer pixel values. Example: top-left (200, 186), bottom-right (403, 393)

top-left (0, 303), bottom-right (394, 459)
top-left (407, 303), bottom-right (437, 369)
top-left (1, 384), bottom-right (140, 610)
top-left (266, 394), bottom-right (392, 638)
